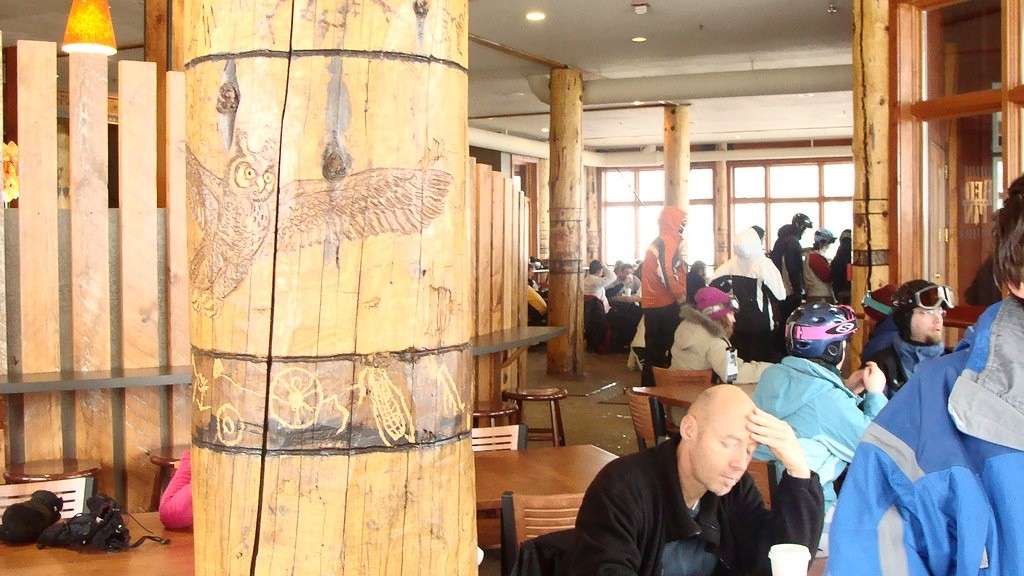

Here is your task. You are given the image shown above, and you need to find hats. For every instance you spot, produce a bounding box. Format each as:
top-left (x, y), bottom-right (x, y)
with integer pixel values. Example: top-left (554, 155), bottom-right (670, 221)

top-left (695, 287), bottom-right (733, 319)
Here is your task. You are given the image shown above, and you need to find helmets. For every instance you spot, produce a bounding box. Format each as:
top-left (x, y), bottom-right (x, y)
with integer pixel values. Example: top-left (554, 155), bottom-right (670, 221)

top-left (840, 229), bottom-right (851, 248)
top-left (814, 228), bottom-right (835, 244)
top-left (786, 301), bottom-right (858, 367)
top-left (891, 278), bottom-right (945, 308)
top-left (792, 213), bottom-right (812, 230)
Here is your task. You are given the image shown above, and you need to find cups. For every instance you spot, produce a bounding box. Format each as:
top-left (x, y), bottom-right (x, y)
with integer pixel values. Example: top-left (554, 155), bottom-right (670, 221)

top-left (768, 544), bottom-right (812, 576)
top-left (625, 288), bottom-right (631, 296)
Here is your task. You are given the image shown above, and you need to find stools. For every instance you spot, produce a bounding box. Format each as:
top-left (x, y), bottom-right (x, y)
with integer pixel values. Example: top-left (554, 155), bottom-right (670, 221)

top-left (506, 385), bottom-right (569, 447)
top-left (3, 458), bottom-right (103, 498)
top-left (148, 442), bottom-right (192, 513)
top-left (472, 400), bottom-right (519, 447)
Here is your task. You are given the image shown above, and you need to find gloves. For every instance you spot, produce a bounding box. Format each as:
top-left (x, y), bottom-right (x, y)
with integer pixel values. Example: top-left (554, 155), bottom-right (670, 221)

top-left (0, 490), bottom-right (64, 544)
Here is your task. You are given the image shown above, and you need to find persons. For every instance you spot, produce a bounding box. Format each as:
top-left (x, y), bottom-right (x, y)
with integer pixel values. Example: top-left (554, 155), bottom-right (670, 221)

top-left (160, 447), bottom-right (192, 533)
top-left (528, 206), bottom-right (958, 576)
top-left (818, 171), bottom-right (1024, 576)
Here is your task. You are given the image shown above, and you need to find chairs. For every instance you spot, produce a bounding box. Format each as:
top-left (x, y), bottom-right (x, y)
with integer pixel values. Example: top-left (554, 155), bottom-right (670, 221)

top-left (0, 476), bottom-right (88, 524)
top-left (623, 384), bottom-right (667, 452)
top-left (500, 488), bottom-right (586, 576)
top-left (746, 458), bottom-right (778, 512)
top-left (582, 292), bottom-right (627, 356)
top-left (472, 423), bottom-right (529, 452)
top-left (651, 365), bottom-right (717, 438)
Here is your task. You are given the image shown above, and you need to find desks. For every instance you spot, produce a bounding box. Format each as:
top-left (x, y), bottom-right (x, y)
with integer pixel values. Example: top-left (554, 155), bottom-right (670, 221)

top-left (474, 443), bottom-right (623, 551)
top-left (608, 293), bottom-right (644, 354)
top-left (635, 380), bottom-right (761, 412)
top-left (0, 511), bottom-right (195, 576)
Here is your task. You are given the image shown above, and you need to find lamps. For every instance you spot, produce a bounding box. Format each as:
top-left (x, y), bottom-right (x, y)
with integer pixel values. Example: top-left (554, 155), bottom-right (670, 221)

top-left (60, 0), bottom-right (118, 56)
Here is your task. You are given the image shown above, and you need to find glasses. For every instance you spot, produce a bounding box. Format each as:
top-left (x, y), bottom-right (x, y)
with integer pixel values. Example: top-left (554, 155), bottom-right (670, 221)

top-left (914, 285), bottom-right (956, 309)
top-left (730, 295), bottom-right (739, 312)
